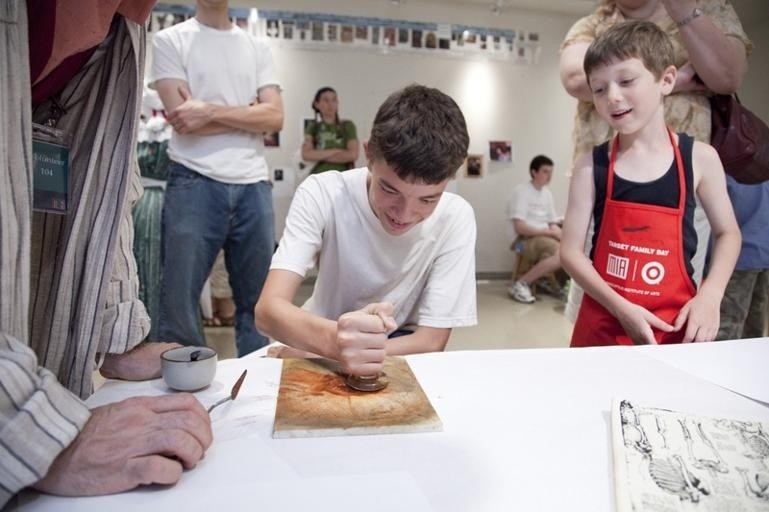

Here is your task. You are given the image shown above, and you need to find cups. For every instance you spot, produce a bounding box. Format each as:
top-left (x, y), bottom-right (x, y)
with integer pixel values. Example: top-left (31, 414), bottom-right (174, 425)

top-left (161, 345), bottom-right (218, 392)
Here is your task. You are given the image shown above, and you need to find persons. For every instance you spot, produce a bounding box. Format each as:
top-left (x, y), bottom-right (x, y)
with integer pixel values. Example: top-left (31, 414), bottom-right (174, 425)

top-left (148, 0), bottom-right (284, 359)
top-left (0, 0), bottom-right (213, 510)
top-left (704, 173), bottom-right (769, 341)
top-left (559, 21), bottom-right (742, 347)
top-left (301, 88), bottom-right (359, 174)
top-left (507, 156), bottom-right (564, 303)
top-left (254, 86), bottom-right (479, 377)
top-left (559, 0), bottom-right (754, 324)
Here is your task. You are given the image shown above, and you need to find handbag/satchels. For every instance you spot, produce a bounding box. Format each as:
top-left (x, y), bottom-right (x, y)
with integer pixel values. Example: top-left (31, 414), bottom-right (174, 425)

top-left (711, 88), bottom-right (768, 184)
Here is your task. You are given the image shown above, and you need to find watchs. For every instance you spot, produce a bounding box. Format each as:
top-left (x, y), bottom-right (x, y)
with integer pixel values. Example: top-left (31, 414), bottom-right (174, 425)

top-left (676, 8), bottom-right (703, 28)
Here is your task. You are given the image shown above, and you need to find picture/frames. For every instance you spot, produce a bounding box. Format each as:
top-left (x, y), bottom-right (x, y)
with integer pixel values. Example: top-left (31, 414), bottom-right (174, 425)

top-left (464, 154), bottom-right (483, 178)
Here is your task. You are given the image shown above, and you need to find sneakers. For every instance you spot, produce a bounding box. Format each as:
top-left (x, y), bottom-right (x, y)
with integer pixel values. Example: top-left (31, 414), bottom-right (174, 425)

top-left (506, 281), bottom-right (561, 303)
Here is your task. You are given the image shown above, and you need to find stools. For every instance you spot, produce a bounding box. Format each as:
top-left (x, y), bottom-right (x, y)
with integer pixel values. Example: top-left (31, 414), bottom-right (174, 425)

top-left (512, 252), bottom-right (561, 301)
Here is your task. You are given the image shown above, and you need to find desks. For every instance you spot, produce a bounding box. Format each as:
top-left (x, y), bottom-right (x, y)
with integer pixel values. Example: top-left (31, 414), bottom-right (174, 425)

top-left (9, 337), bottom-right (769, 512)
